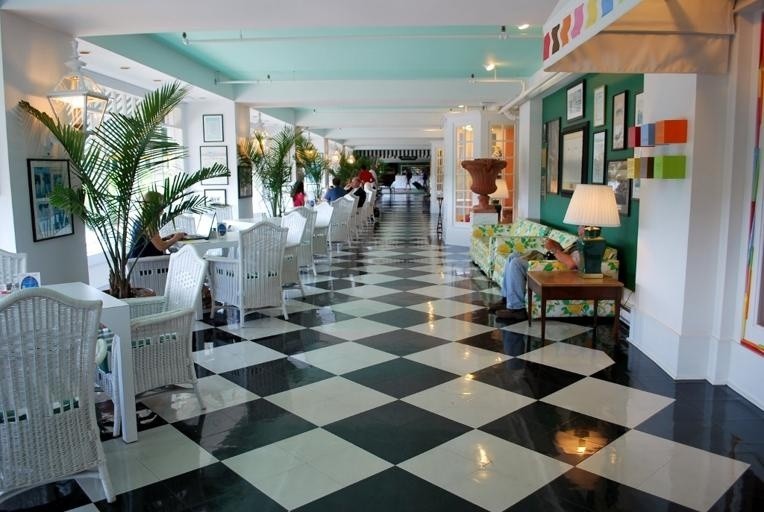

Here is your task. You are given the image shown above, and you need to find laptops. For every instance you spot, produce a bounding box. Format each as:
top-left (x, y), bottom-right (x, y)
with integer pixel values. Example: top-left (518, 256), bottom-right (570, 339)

top-left (177, 211), bottom-right (216, 241)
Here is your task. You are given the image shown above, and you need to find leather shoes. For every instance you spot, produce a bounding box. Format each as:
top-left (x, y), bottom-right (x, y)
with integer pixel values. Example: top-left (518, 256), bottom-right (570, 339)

top-left (487, 298), bottom-right (528, 320)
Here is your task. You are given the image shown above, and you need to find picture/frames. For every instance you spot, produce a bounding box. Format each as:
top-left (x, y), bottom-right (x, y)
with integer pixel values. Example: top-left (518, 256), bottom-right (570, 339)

top-left (203, 187), bottom-right (227, 209)
top-left (200, 145), bottom-right (229, 187)
top-left (284, 167), bottom-right (291, 182)
top-left (202, 113), bottom-right (225, 143)
top-left (26, 154), bottom-right (75, 244)
top-left (536, 76), bottom-right (641, 221)
top-left (237, 165), bottom-right (253, 199)
top-left (269, 148), bottom-right (290, 164)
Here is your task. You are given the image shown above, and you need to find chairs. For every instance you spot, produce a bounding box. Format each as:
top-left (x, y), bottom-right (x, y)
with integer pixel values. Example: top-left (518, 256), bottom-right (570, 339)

top-left (95, 242), bottom-right (209, 438)
top-left (208, 182), bottom-right (381, 330)
top-left (126, 254), bottom-right (204, 321)
top-left (2, 285), bottom-right (118, 506)
top-left (0, 246), bottom-right (29, 293)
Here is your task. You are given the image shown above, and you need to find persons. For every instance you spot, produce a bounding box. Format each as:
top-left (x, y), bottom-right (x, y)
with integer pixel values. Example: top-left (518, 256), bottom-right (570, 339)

top-left (127, 190), bottom-right (212, 310)
top-left (488, 225), bottom-right (602, 323)
top-left (323, 162), bottom-right (379, 209)
top-left (289, 182), bottom-right (308, 208)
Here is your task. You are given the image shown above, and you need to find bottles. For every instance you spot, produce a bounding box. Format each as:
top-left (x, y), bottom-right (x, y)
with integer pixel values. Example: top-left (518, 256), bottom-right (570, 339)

top-left (209, 228), bottom-right (217, 241)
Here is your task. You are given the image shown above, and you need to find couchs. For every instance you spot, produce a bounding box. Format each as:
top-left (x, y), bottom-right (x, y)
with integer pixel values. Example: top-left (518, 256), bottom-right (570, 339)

top-left (491, 222), bottom-right (621, 320)
top-left (469, 216), bottom-right (551, 280)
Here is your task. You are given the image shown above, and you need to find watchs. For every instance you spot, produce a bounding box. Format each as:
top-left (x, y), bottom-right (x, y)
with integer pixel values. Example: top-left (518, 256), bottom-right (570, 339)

top-left (548, 246), bottom-right (557, 255)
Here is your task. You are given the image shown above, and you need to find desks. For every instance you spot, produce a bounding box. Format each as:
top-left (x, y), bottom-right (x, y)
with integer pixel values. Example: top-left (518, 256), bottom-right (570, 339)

top-left (436, 195), bottom-right (443, 218)
top-left (35, 281), bottom-right (140, 445)
top-left (517, 265), bottom-right (629, 348)
top-left (159, 205), bottom-right (219, 237)
top-left (161, 235), bottom-right (239, 258)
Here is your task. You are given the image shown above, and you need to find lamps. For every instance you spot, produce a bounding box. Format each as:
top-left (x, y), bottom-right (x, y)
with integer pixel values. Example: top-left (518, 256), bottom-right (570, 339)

top-left (44, 35), bottom-right (112, 151)
top-left (487, 176), bottom-right (512, 221)
top-left (559, 182), bottom-right (625, 281)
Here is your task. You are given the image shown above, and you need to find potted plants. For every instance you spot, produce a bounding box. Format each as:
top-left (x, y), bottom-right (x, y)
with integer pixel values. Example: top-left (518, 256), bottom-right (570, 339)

top-left (13, 75), bottom-right (232, 300)
